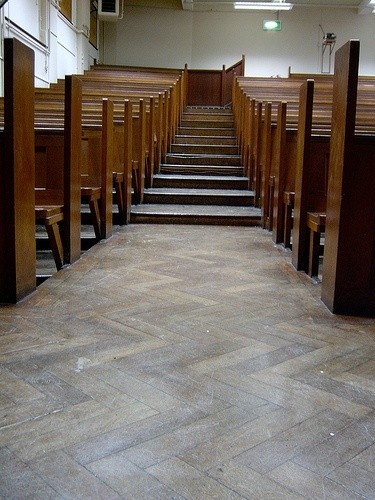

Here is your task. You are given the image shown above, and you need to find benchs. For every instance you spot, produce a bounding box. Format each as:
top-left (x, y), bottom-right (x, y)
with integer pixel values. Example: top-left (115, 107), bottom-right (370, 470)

top-left (0, 37), bottom-right (186, 304)
top-left (232, 38), bottom-right (375, 319)
